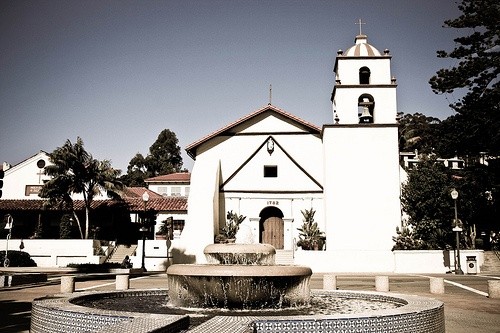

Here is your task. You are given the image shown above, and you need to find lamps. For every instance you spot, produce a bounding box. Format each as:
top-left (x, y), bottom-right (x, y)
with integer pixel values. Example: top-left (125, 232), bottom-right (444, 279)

top-left (267, 138), bottom-right (274, 156)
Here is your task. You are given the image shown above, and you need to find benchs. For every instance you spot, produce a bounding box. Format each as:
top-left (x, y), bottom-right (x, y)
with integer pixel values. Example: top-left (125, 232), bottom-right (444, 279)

top-left (0, 266), bottom-right (77, 287)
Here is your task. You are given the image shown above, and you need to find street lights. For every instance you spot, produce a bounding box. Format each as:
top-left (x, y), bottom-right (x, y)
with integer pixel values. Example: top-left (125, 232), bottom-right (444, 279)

top-left (450, 188), bottom-right (464, 274)
top-left (141, 190), bottom-right (149, 273)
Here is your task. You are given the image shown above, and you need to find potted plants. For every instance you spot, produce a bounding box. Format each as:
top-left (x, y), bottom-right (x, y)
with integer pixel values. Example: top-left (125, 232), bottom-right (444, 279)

top-left (215, 209), bottom-right (245, 243)
top-left (297, 208), bottom-right (324, 249)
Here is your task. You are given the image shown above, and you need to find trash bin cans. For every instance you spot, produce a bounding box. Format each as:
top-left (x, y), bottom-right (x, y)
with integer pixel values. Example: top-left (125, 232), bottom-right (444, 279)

top-left (465, 256), bottom-right (477, 275)
